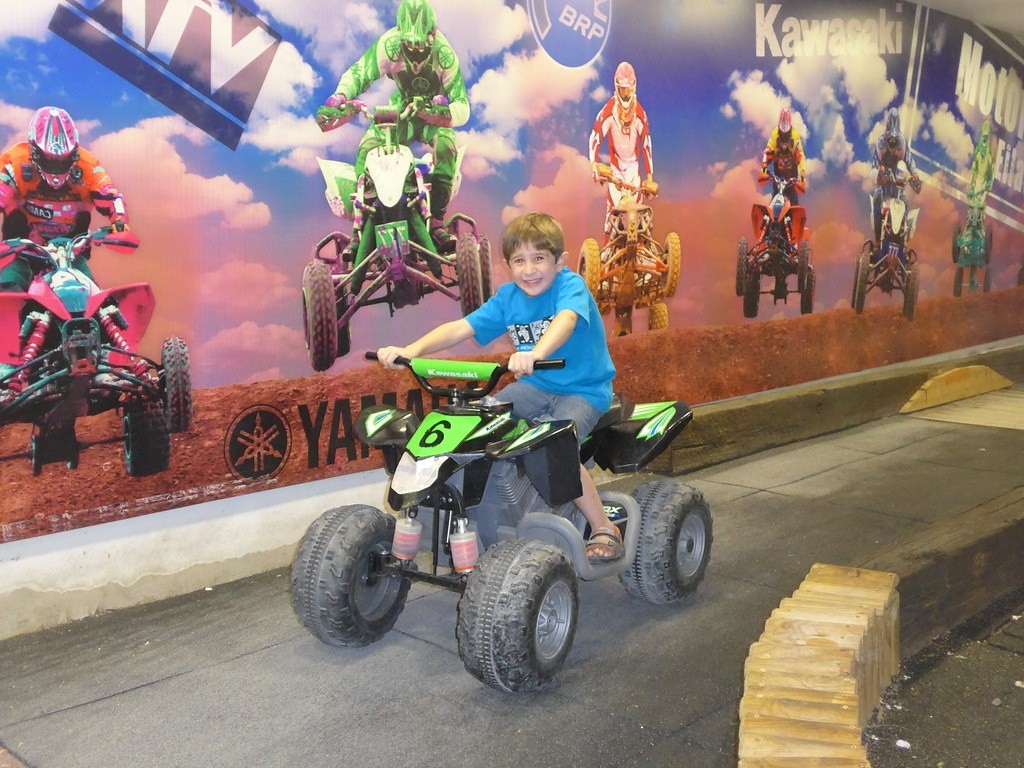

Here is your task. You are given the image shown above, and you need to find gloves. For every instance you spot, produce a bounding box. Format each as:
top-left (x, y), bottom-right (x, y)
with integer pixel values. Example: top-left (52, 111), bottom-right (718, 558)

top-left (642, 182), bottom-right (658, 200)
top-left (111, 222), bottom-right (129, 235)
top-left (432, 96), bottom-right (448, 108)
top-left (325, 94), bottom-right (347, 109)
top-left (594, 169), bottom-right (609, 184)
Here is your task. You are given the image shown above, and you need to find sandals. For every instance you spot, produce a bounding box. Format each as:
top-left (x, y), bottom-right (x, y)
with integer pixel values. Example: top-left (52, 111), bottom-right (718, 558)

top-left (585, 527), bottom-right (625, 565)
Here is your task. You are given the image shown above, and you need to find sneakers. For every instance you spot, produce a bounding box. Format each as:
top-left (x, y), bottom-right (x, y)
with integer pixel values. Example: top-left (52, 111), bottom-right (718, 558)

top-left (432, 226), bottom-right (457, 257)
top-left (342, 235), bottom-right (361, 263)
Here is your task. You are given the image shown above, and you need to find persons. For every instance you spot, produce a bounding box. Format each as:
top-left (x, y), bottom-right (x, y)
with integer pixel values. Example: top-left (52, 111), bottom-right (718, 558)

top-left (325, 0), bottom-right (470, 260)
top-left (871, 108), bottom-right (922, 262)
top-left (0, 102), bottom-right (129, 395)
top-left (377, 212), bottom-right (625, 560)
top-left (759, 108), bottom-right (807, 208)
top-left (588, 62), bottom-right (660, 290)
top-left (966, 117), bottom-right (993, 230)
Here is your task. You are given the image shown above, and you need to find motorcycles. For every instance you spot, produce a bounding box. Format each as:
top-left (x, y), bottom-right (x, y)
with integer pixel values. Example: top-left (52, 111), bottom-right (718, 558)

top-left (285, 349), bottom-right (714, 697)
top-left (301, 92), bottom-right (500, 369)
top-left (848, 167), bottom-right (922, 319)
top-left (733, 168), bottom-right (816, 318)
top-left (578, 168), bottom-right (679, 338)
top-left (0, 227), bottom-right (190, 476)
top-left (952, 184), bottom-right (994, 300)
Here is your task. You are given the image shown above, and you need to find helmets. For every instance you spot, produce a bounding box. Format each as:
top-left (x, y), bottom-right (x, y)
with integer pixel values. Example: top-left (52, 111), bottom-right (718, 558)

top-left (396, 0), bottom-right (435, 73)
top-left (614, 61), bottom-right (636, 124)
top-left (886, 108), bottom-right (902, 153)
top-left (981, 120), bottom-right (989, 156)
top-left (779, 109), bottom-right (792, 151)
top-left (29, 107), bottom-right (79, 188)
